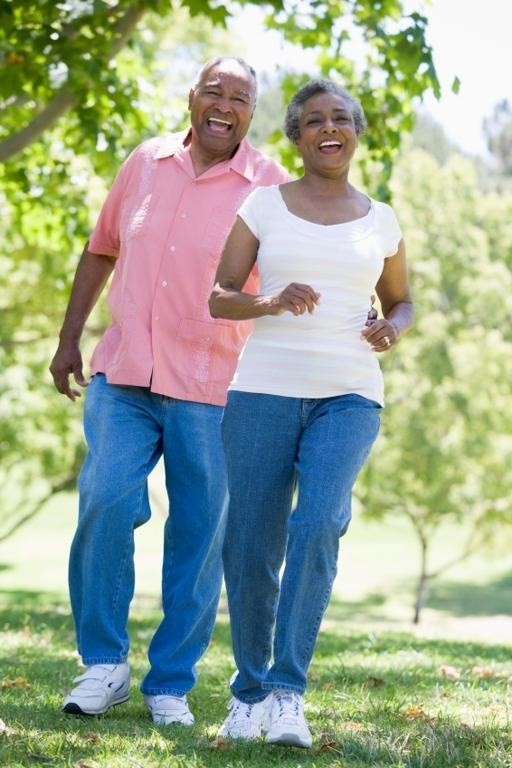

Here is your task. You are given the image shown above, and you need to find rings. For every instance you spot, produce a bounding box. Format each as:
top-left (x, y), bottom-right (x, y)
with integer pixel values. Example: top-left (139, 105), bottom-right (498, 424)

top-left (382, 336), bottom-right (391, 348)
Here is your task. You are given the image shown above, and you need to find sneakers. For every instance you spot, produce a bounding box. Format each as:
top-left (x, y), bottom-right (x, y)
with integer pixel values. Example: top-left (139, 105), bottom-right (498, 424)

top-left (141, 691), bottom-right (196, 727)
top-left (63, 660), bottom-right (131, 716)
top-left (216, 694), bottom-right (273, 741)
top-left (265, 688), bottom-right (314, 748)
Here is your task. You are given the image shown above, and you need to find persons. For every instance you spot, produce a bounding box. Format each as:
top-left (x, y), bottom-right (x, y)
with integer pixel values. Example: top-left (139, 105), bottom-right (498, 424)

top-left (47, 52), bottom-right (299, 730)
top-left (205, 75), bottom-right (420, 750)
